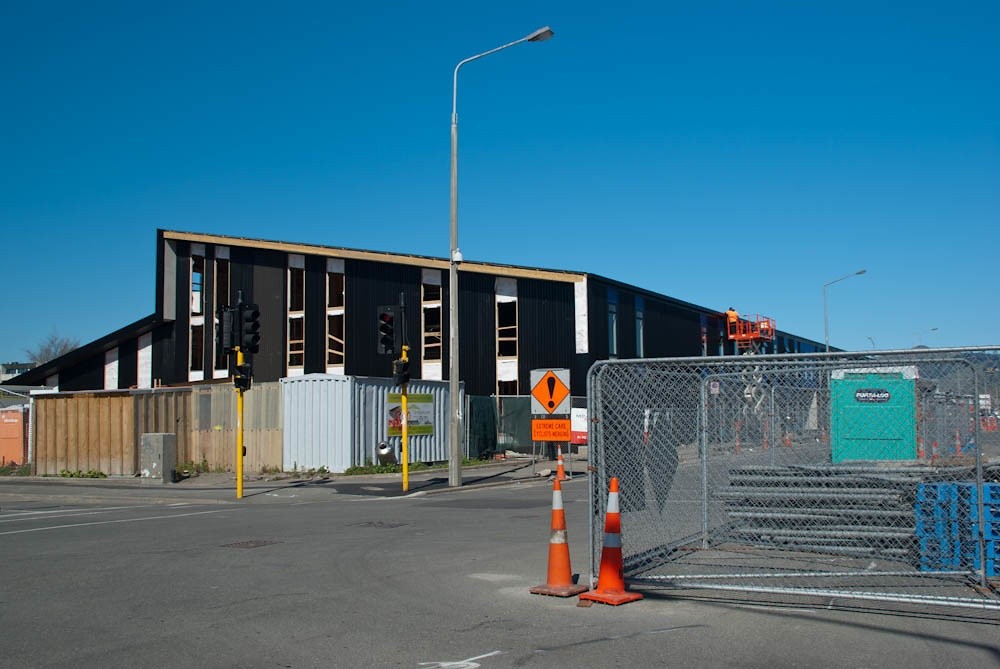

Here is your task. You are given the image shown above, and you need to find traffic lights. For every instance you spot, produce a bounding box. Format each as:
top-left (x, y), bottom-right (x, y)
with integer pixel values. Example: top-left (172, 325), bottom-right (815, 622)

top-left (233, 302), bottom-right (261, 355)
top-left (230, 361), bottom-right (252, 391)
top-left (392, 360), bottom-right (410, 386)
top-left (376, 304), bottom-right (402, 358)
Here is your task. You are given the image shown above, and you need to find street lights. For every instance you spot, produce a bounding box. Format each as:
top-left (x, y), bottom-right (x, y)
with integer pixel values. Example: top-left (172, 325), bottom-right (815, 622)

top-left (823, 269), bottom-right (869, 451)
top-left (447, 23), bottom-right (555, 487)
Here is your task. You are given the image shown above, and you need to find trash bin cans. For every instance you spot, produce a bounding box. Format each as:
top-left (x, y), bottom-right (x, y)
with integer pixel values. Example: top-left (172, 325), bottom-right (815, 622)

top-left (376, 442), bottom-right (397, 465)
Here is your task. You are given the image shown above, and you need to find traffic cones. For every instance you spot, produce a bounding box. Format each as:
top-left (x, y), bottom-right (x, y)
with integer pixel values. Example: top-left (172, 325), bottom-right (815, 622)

top-left (552, 445), bottom-right (571, 481)
top-left (785, 430), bottom-right (792, 447)
top-left (527, 478), bottom-right (597, 598)
top-left (577, 478), bottom-right (644, 607)
